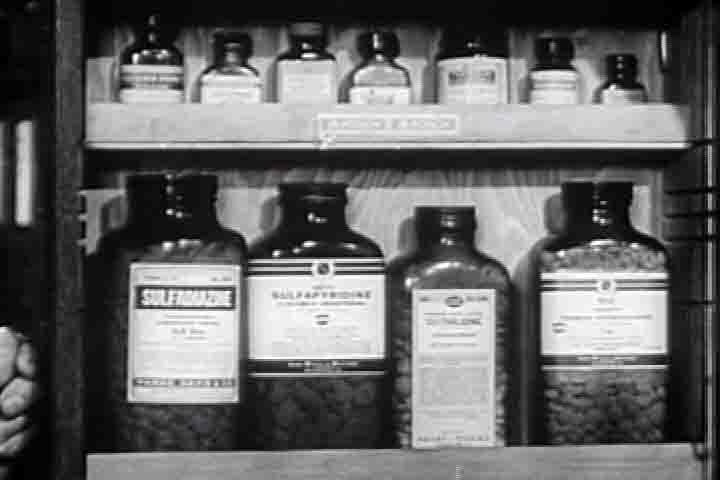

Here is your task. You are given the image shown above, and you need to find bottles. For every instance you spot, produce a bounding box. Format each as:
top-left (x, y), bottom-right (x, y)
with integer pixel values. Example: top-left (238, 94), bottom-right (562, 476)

top-left (99, 174), bottom-right (246, 450)
top-left (273, 20), bottom-right (340, 103)
top-left (247, 181), bottom-right (389, 451)
top-left (198, 33), bottom-right (263, 101)
top-left (390, 206), bottom-right (514, 445)
top-left (530, 180), bottom-right (672, 445)
top-left (345, 33), bottom-right (412, 105)
top-left (528, 38), bottom-right (582, 103)
top-left (434, 23), bottom-right (510, 103)
top-left (118, 15), bottom-right (186, 103)
top-left (595, 54), bottom-right (646, 103)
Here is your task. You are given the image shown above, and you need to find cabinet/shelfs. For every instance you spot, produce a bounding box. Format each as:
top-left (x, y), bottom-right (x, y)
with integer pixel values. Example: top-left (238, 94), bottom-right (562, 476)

top-left (56, 0), bottom-right (712, 478)
